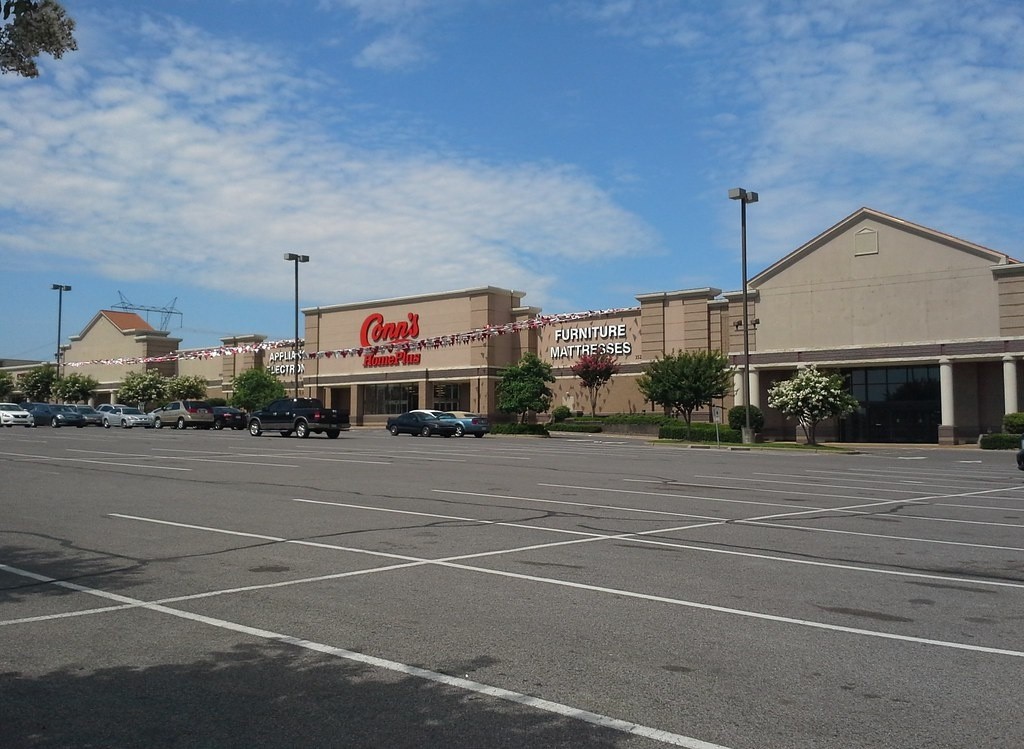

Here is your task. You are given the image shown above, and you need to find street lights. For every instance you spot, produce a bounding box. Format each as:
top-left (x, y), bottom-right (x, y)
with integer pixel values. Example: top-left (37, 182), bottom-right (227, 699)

top-left (283, 252), bottom-right (309, 396)
top-left (50, 283), bottom-right (72, 407)
top-left (728, 186), bottom-right (760, 446)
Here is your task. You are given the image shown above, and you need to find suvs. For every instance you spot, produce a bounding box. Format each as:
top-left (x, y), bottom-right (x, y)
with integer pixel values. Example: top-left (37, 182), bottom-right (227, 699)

top-left (152, 400), bottom-right (215, 429)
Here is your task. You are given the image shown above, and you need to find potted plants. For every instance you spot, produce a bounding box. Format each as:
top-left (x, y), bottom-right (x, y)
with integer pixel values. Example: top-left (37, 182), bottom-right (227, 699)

top-left (727, 405), bottom-right (765, 444)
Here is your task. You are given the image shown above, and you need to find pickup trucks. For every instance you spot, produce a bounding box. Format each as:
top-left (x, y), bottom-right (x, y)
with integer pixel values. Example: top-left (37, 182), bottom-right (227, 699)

top-left (246, 397), bottom-right (351, 439)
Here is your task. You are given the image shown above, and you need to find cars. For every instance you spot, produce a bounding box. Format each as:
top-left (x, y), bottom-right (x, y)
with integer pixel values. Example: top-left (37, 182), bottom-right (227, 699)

top-left (147, 406), bottom-right (171, 428)
top-left (419, 409), bottom-right (492, 437)
top-left (19, 401), bottom-right (126, 428)
top-left (195, 405), bottom-right (246, 431)
top-left (408, 409), bottom-right (449, 419)
top-left (101, 406), bottom-right (152, 429)
top-left (384, 410), bottom-right (454, 436)
top-left (0, 403), bottom-right (35, 429)
